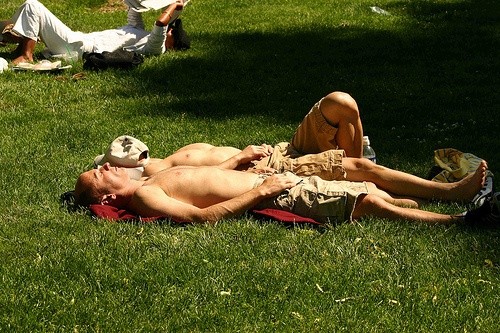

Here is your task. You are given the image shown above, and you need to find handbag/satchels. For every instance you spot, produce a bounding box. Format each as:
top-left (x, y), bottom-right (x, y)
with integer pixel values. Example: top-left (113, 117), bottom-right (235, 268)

top-left (82, 46), bottom-right (146, 72)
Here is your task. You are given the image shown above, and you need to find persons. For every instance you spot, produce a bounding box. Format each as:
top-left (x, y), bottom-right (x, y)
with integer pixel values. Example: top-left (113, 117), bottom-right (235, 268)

top-left (93, 92), bottom-right (487, 202)
top-left (75, 162), bottom-right (500, 224)
top-left (10, 0), bottom-right (191, 65)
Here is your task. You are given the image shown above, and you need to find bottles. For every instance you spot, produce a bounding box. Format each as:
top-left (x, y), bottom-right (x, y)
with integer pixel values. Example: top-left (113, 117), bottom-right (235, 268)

top-left (361, 136), bottom-right (376, 165)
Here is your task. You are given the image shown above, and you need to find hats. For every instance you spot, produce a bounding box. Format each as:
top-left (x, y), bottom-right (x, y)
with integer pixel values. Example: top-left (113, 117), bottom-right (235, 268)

top-left (92, 134), bottom-right (150, 167)
top-left (173, 18), bottom-right (190, 49)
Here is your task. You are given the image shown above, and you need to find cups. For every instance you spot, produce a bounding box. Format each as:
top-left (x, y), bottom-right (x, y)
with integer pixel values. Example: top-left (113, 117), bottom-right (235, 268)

top-left (63, 51), bottom-right (79, 62)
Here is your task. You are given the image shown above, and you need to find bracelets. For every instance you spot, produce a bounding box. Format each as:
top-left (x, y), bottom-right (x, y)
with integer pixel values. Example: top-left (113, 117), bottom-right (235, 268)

top-left (164, 11), bottom-right (172, 19)
